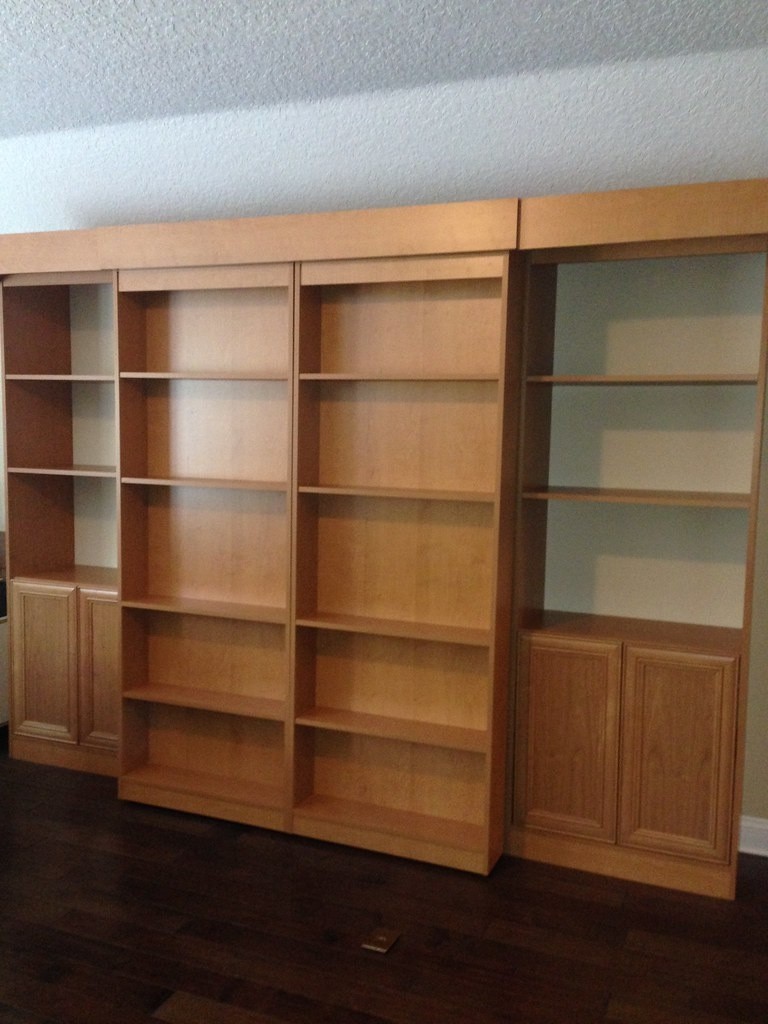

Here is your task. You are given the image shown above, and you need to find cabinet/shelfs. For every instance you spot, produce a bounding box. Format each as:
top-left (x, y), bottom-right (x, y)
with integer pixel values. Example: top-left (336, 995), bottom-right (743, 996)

top-left (0, 180), bottom-right (768, 901)
